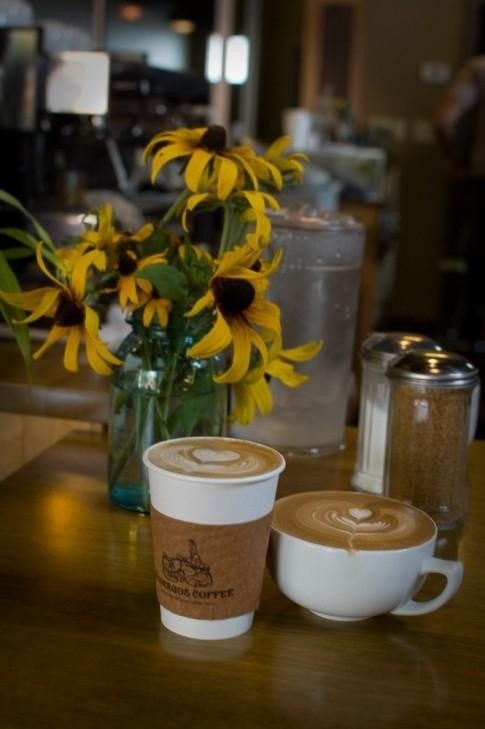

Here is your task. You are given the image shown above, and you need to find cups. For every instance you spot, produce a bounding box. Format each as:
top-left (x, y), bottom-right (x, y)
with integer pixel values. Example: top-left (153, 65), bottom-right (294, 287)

top-left (142, 438), bottom-right (286, 640)
top-left (265, 489), bottom-right (468, 624)
top-left (231, 208), bottom-right (365, 455)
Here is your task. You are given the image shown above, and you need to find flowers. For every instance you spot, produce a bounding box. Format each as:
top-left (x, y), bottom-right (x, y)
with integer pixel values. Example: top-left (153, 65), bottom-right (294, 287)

top-left (0, 121), bottom-right (324, 506)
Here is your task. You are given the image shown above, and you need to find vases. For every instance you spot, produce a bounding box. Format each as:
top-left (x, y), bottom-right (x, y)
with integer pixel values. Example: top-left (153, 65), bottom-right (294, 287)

top-left (105, 314), bottom-right (231, 514)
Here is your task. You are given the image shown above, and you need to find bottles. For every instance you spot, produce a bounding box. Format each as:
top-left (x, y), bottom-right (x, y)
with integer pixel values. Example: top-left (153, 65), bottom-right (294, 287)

top-left (351, 334), bottom-right (441, 495)
top-left (385, 349), bottom-right (477, 529)
